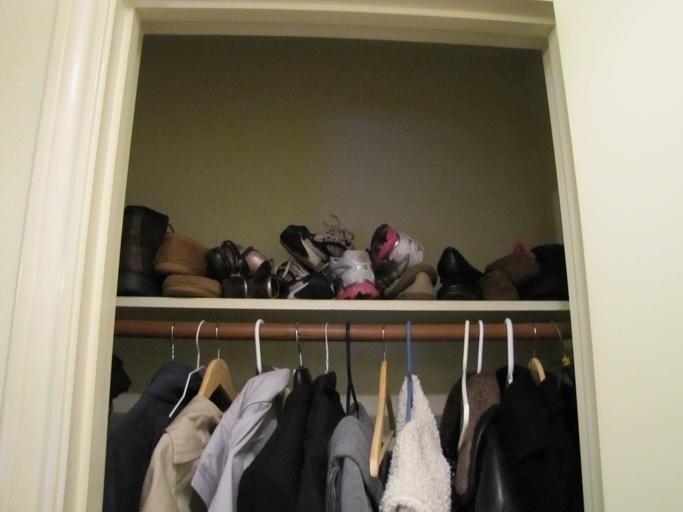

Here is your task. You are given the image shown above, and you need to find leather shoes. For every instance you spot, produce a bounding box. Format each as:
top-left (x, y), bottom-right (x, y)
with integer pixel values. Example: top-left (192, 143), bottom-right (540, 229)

top-left (438, 246), bottom-right (482, 283)
top-left (438, 279), bottom-right (480, 300)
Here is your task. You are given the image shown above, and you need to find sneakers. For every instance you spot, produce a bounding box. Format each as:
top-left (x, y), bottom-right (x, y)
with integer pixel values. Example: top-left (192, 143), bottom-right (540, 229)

top-left (206, 239), bottom-right (244, 277)
top-left (280, 224), bottom-right (353, 280)
top-left (371, 224), bottom-right (425, 285)
top-left (327, 248), bottom-right (380, 300)
top-left (243, 246), bottom-right (274, 278)
top-left (478, 268), bottom-right (519, 300)
top-left (161, 272), bottom-right (223, 297)
top-left (277, 260), bottom-right (336, 298)
top-left (485, 253), bottom-right (541, 290)
top-left (220, 273), bottom-right (255, 298)
top-left (154, 232), bottom-right (217, 277)
top-left (254, 275), bottom-right (281, 298)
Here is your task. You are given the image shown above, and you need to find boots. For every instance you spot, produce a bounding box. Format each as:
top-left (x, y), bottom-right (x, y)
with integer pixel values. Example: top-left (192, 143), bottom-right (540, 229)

top-left (523, 244), bottom-right (566, 298)
top-left (118, 205), bottom-right (169, 295)
top-left (384, 262), bottom-right (437, 300)
